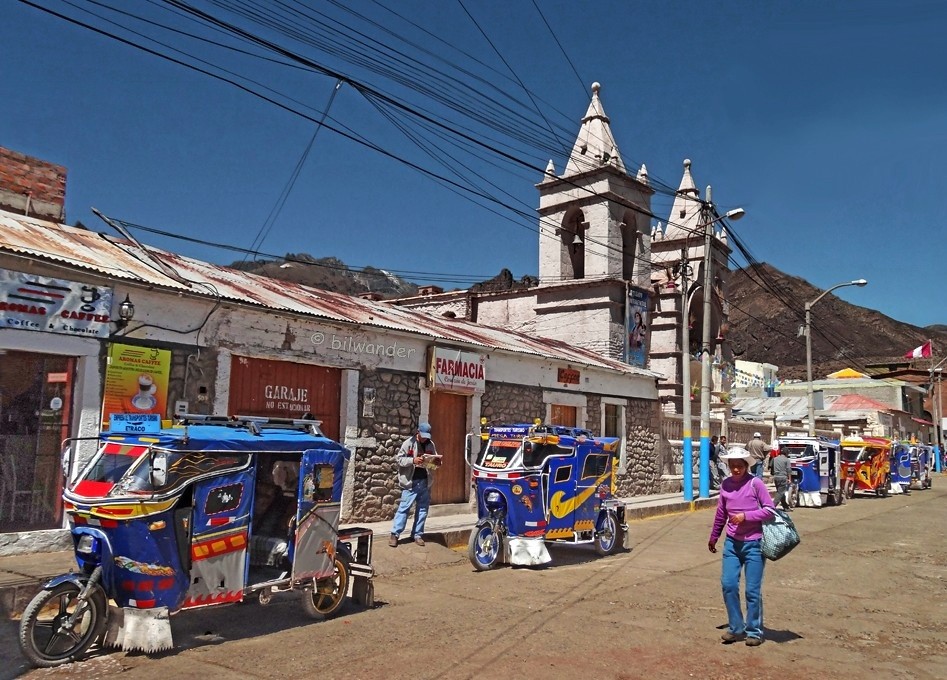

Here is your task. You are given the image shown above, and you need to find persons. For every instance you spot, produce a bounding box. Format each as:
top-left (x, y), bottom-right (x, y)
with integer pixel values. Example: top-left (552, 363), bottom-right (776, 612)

top-left (715, 435), bottom-right (730, 480)
top-left (745, 432), bottom-right (771, 481)
top-left (770, 447), bottom-right (794, 512)
top-left (707, 446), bottom-right (777, 646)
top-left (708, 436), bottom-right (721, 490)
top-left (388, 423), bottom-right (442, 548)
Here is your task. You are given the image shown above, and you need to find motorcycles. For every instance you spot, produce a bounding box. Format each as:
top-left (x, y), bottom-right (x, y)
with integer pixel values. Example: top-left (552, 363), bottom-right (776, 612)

top-left (777, 430), bottom-right (843, 511)
top-left (839, 432), bottom-right (893, 500)
top-left (904, 441), bottom-right (932, 494)
top-left (463, 416), bottom-right (629, 570)
top-left (873, 439), bottom-right (911, 498)
top-left (17, 406), bottom-right (350, 665)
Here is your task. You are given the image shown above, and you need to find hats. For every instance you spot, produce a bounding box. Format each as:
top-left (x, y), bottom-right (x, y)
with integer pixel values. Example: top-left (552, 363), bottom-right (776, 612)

top-left (781, 448), bottom-right (790, 453)
top-left (753, 432), bottom-right (761, 437)
top-left (720, 446), bottom-right (757, 466)
top-left (418, 422), bottom-right (432, 439)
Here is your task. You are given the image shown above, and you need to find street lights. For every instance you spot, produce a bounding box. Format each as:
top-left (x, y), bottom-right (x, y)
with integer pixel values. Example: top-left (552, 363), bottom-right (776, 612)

top-left (679, 208), bottom-right (746, 501)
top-left (805, 278), bottom-right (868, 436)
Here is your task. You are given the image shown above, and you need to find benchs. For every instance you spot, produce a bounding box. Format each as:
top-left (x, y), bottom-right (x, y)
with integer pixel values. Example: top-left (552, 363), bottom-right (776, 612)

top-left (250, 482), bottom-right (300, 570)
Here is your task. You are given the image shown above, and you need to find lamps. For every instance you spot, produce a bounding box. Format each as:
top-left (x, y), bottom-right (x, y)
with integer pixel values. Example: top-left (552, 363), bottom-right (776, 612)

top-left (108, 292), bottom-right (134, 339)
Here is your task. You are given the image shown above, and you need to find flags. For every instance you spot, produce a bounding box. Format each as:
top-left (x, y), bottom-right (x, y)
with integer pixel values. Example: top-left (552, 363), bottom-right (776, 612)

top-left (903, 339), bottom-right (931, 359)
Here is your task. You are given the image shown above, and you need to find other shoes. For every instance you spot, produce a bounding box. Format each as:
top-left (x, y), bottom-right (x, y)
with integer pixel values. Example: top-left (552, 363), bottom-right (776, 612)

top-left (388, 534), bottom-right (398, 547)
top-left (412, 537), bottom-right (425, 546)
top-left (785, 507), bottom-right (794, 512)
top-left (721, 630), bottom-right (747, 642)
top-left (746, 637), bottom-right (761, 646)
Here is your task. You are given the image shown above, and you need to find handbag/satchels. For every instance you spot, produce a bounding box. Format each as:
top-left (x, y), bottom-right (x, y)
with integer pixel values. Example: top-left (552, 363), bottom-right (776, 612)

top-left (751, 478), bottom-right (800, 561)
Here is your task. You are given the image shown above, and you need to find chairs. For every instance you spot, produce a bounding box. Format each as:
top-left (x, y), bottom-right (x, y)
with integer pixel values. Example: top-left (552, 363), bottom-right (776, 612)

top-left (0, 454), bottom-right (42, 524)
